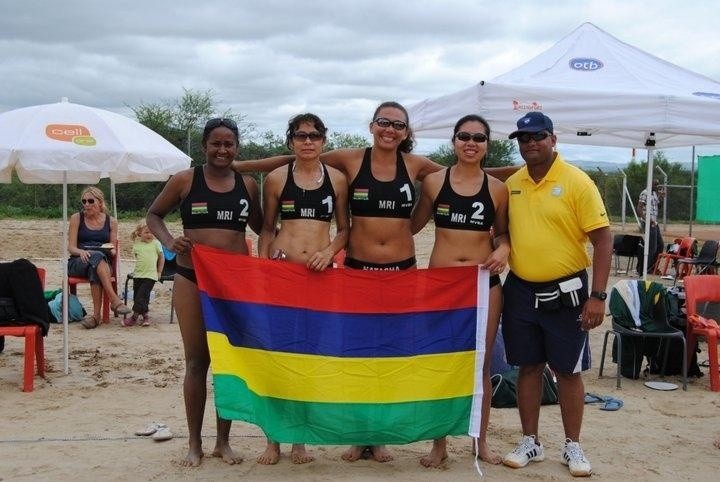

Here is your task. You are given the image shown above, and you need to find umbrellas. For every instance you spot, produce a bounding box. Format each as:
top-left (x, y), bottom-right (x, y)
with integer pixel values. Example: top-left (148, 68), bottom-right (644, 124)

top-left (1, 96), bottom-right (193, 376)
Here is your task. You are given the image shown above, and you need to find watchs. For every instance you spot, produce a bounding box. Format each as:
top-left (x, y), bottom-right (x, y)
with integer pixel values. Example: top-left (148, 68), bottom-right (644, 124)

top-left (590, 289), bottom-right (608, 300)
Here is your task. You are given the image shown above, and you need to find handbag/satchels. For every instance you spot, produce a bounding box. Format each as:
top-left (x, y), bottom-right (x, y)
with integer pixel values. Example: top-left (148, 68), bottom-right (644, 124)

top-left (490, 366), bottom-right (558, 408)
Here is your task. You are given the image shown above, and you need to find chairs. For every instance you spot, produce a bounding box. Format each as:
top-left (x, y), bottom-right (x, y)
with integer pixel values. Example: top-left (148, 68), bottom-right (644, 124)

top-left (1, 262), bottom-right (47, 393)
top-left (123, 242), bottom-right (176, 324)
top-left (597, 279), bottom-right (688, 395)
top-left (680, 274), bottom-right (720, 392)
top-left (613, 227), bottom-right (717, 272)
top-left (62, 236), bottom-right (118, 327)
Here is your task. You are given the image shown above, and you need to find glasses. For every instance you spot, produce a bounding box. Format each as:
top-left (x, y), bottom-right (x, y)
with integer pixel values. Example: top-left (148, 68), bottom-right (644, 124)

top-left (81, 199), bottom-right (95, 204)
top-left (454, 131), bottom-right (488, 143)
top-left (372, 117), bottom-right (408, 131)
top-left (292, 130), bottom-right (324, 141)
top-left (518, 132), bottom-right (553, 142)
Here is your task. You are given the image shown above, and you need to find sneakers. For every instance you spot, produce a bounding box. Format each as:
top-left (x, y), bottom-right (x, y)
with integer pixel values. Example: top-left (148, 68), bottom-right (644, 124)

top-left (143, 318), bottom-right (151, 325)
top-left (81, 317), bottom-right (99, 329)
top-left (111, 302), bottom-right (131, 314)
top-left (121, 317), bottom-right (137, 326)
top-left (560, 438), bottom-right (592, 477)
top-left (502, 433), bottom-right (545, 468)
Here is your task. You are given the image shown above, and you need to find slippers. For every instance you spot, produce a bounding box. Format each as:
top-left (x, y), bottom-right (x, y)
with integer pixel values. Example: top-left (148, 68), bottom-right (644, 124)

top-left (152, 426), bottom-right (172, 440)
top-left (135, 422), bottom-right (165, 435)
top-left (600, 399), bottom-right (624, 411)
top-left (583, 392), bottom-right (614, 403)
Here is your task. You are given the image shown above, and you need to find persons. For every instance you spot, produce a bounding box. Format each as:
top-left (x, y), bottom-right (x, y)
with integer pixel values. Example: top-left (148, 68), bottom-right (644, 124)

top-left (497, 113), bottom-right (615, 476)
top-left (413, 110), bottom-right (509, 467)
top-left (65, 186), bottom-right (131, 329)
top-left (255, 110), bottom-right (349, 467)
top-left (232, 100), bottom-right (529, 466)
top-left (121, 219), bottom-right (167, 328)
top-left (138, 118), bottom-right (246, 468)
top-left (636, 178), bottom-right (667, 277)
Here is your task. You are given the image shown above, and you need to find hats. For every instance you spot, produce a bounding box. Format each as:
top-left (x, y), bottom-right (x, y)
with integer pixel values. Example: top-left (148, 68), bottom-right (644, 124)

top-left (509, 112), bottom-right (553, 139)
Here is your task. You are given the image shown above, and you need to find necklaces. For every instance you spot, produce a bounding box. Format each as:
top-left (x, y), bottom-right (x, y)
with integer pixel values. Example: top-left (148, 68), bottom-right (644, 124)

top-left (531, 176), bottom-right (544, 182)
top-left (292, 160), bottom-right (325, 198)
